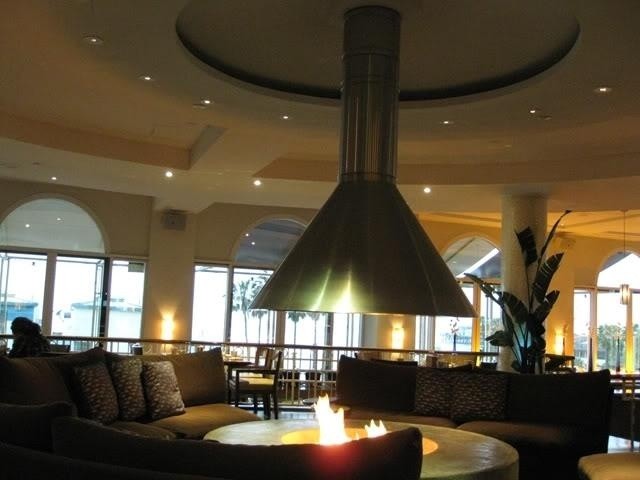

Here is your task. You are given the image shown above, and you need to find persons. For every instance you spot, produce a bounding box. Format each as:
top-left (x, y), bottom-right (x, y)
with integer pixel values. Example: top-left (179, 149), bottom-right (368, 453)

top-left (5, 317), bottom-right (50, 359)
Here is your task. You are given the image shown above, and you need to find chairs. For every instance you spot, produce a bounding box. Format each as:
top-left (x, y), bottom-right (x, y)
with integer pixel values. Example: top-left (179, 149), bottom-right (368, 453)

top-left (227, 348), bottom-right (284, 419)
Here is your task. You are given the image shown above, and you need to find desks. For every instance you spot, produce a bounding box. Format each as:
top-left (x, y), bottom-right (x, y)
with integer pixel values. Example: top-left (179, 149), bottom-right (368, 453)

top-left (224, 361), bottom-right (252, 367)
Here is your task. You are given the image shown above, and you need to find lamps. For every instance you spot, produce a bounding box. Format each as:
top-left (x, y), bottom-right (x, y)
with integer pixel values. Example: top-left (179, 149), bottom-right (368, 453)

top-left (617, 212), bottom-right (631, 307)
top-left (244, 4), bottom-right (482, 323)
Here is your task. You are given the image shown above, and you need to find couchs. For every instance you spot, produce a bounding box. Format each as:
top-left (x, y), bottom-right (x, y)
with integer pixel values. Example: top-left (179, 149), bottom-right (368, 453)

top-left (334, 353), bottom-right (614, 479)
top-left (1, 347), bottom-right (421, 478)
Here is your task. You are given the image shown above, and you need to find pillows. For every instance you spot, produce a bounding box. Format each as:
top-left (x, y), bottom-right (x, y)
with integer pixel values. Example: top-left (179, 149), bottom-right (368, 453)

top-left (142, 358), bottom-right (185, 421)
top-left (109, 356), bottom-right (148, 423)
top-left (408, 364), bottom-right (515, 423)
top-left (58, 358), bottom-right (121, 423)
